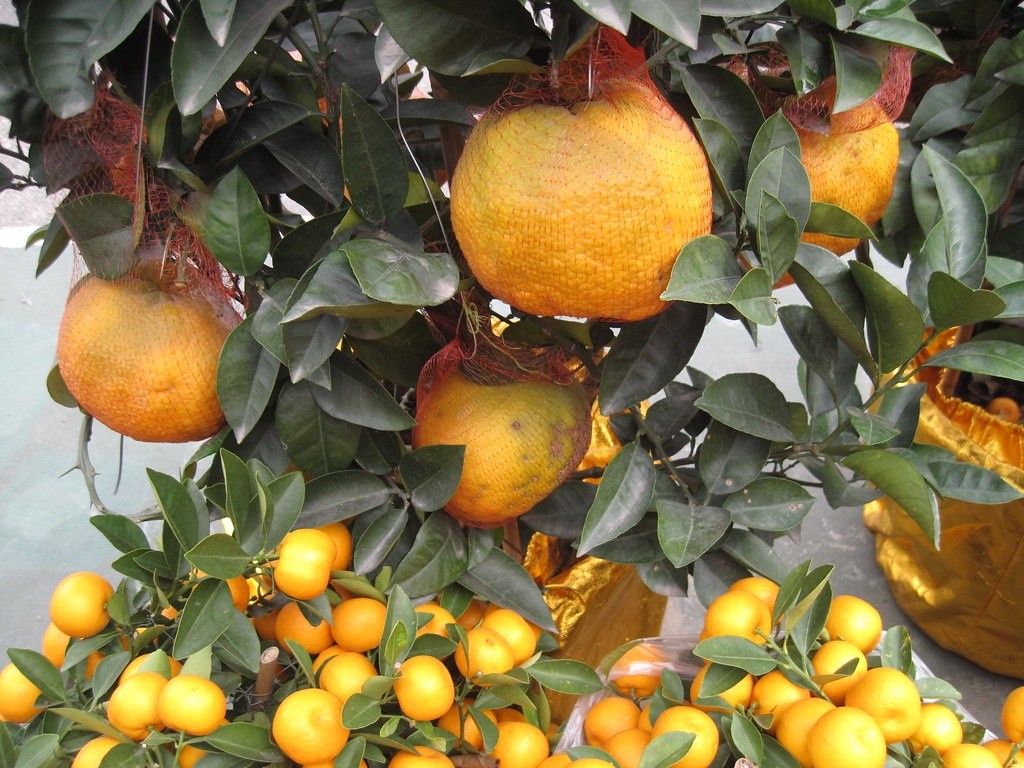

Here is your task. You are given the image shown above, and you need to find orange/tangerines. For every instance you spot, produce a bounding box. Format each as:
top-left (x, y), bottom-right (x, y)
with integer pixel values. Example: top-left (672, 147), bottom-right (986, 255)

top-left (0, 67), bottom-right (1024, 768)
